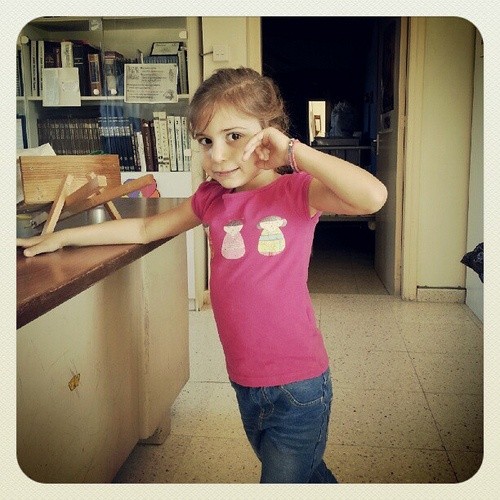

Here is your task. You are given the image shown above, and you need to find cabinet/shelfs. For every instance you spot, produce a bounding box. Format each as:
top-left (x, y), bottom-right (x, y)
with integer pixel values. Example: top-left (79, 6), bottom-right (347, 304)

top-left (316, 137), bottom-right (361, 166)
top-left (16, 15), bottom-right (190, 173)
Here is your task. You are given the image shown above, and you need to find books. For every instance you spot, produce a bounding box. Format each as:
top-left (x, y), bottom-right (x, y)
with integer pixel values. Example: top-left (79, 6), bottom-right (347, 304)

top-left (15, 34), bottom-right (193, 172)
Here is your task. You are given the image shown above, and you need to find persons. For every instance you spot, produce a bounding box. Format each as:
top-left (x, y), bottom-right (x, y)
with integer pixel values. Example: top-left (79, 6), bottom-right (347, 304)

top-left (16, 64), bottom-right (390, 484)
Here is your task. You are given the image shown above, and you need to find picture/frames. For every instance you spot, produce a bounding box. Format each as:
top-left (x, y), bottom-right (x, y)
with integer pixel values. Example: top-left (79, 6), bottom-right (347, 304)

top-left (16, 114), bottom-right (28, 150)
top-left (150, 41), bottom-right (184, 56)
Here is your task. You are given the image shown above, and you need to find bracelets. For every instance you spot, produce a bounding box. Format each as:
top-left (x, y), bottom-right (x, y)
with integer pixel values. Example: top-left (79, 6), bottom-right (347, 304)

top-left (291, 138), bottom-right (303, 174)
top-left (287, 137), bottom-right (296, 173)
